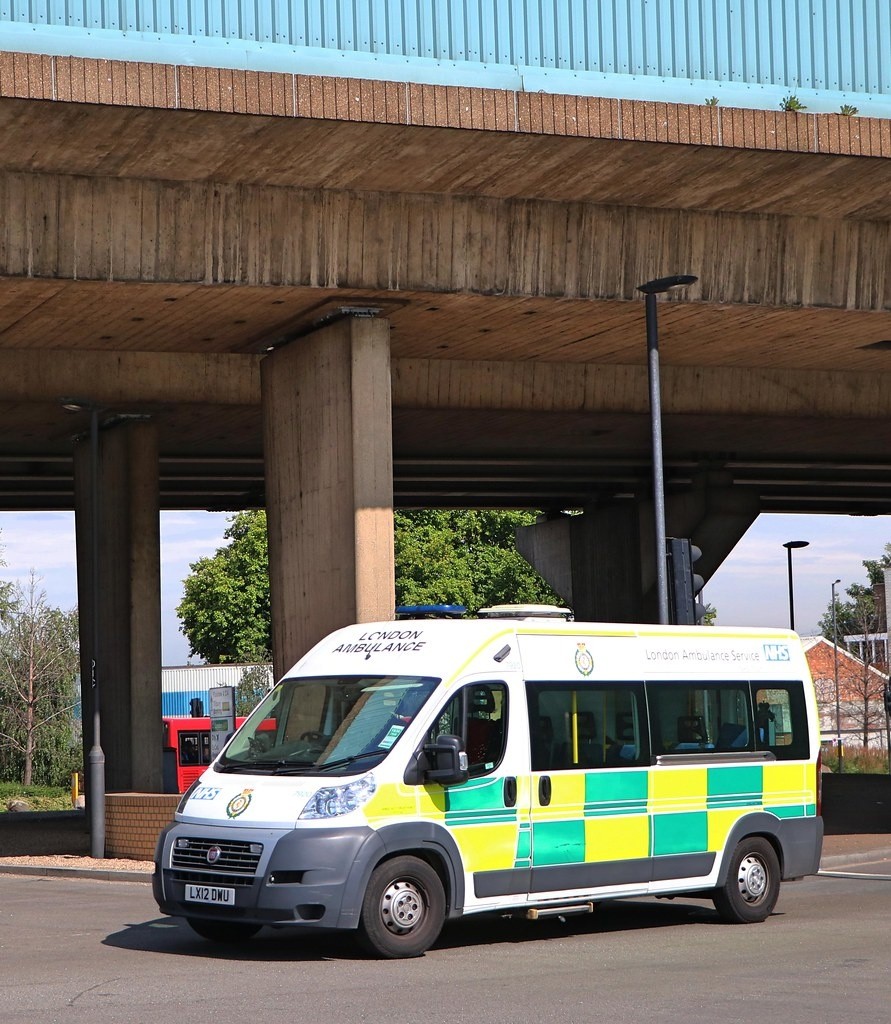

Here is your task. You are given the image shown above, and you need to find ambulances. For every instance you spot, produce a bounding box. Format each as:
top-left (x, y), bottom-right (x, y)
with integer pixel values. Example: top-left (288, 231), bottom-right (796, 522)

top-left (151, 602), bottom-right (826, 960)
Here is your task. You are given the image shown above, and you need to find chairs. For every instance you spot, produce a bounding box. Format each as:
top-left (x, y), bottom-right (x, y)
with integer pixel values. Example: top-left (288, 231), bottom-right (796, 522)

top-left (531, 715), bottom-right (556, 772)
top-left (457, 684), bottom-right (501, 767)
top-left (669, 715), bottom-right (714, 751)
top-left (605, 711), bottom-right (636, 768)
top-left (552, 711), bottom-right (603, 772)
top-left (716, 722), bottom-right (764, 748)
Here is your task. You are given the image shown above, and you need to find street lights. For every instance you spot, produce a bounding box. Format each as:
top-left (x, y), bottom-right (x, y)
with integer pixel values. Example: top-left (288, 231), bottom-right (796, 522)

top-left (783, 539), bottom-right (810, 631)
top-left (635, 273), bottom-right (698, 622)
